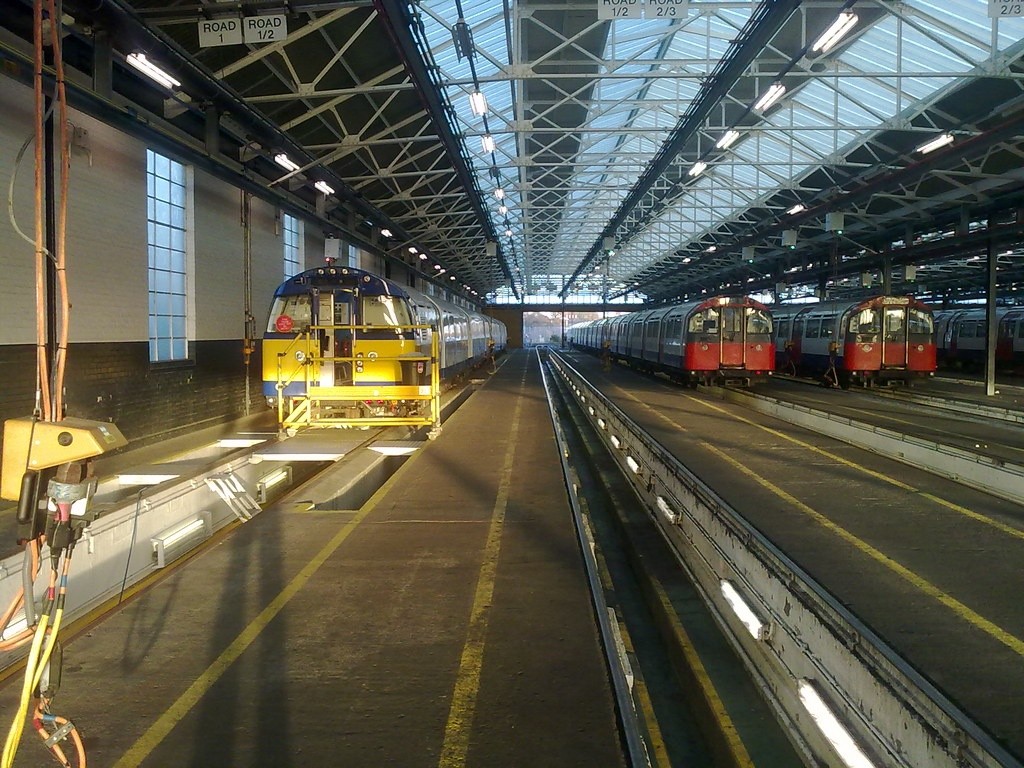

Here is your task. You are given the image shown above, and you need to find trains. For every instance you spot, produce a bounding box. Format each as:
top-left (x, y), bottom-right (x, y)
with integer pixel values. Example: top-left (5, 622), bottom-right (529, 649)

top-left (764, 294), bottom-right (938, 391)
top-left (260, 257), bottom-right (506, 421)
top-left (928, 305), bottom-right (1023, 382)
top-left (566, 292), bottom-right (776, 390)
